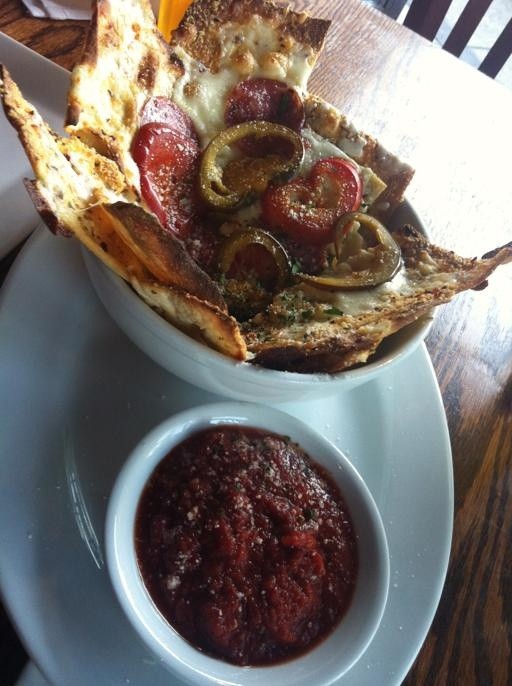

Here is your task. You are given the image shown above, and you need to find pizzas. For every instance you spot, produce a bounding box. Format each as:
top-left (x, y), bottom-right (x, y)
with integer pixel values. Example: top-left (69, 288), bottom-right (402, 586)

top-left (0, 0), bottom-right (512, 374)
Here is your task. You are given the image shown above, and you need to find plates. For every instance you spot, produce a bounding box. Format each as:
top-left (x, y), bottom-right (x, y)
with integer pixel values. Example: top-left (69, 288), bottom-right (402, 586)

top-left (1, 215), bottom-right (459, 685)
top-left (0, 32), bottom-right (70, 262)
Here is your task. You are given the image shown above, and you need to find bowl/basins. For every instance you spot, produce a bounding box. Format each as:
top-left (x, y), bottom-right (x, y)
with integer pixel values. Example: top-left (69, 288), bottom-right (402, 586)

top-left (105, 402), bottom-right (391, 685)
top-left (80, 185), bottom-right (438, 404)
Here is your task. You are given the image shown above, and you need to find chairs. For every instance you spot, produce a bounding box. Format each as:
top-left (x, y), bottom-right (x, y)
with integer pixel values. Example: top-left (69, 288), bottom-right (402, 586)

top-left (372, 0), bottom-right (512, 81)
